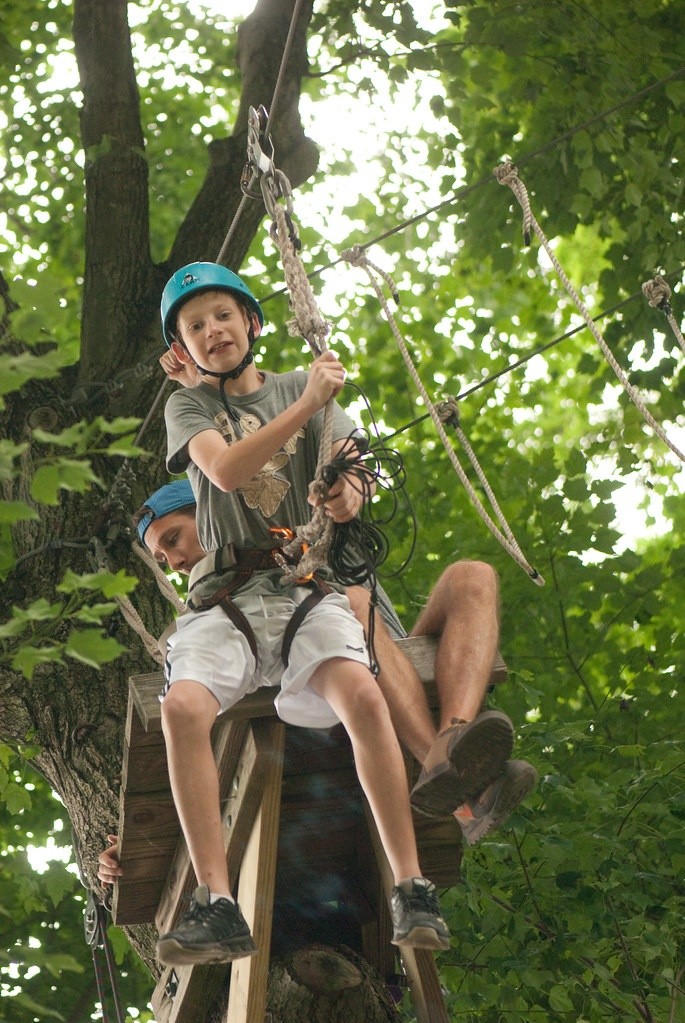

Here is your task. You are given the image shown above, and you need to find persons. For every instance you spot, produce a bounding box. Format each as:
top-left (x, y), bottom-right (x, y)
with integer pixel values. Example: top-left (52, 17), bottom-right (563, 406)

top-left (155, 260), bottom-right (452, 965)
top-left (97, 346), bottom-right (539, 886)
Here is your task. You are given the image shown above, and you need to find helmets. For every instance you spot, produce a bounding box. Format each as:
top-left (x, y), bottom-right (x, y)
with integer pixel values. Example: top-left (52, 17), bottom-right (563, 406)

top-left (160, 262), bottom-right (263, 349)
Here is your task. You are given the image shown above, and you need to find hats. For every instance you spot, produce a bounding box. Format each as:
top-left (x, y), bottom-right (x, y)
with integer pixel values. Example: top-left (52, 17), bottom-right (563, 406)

top-left (139, 479), bottom-right (196, 546)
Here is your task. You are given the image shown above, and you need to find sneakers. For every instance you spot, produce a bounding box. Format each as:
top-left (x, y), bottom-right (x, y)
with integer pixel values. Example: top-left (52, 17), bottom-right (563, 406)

top-left (391, 876), bottom-right (451, 951)
top-left (454, 761), bottom-right (538, 847)
top-left (156, 883), bottom-right (259, 967)
top-left (408, 710), bottom-right (513, 820)
